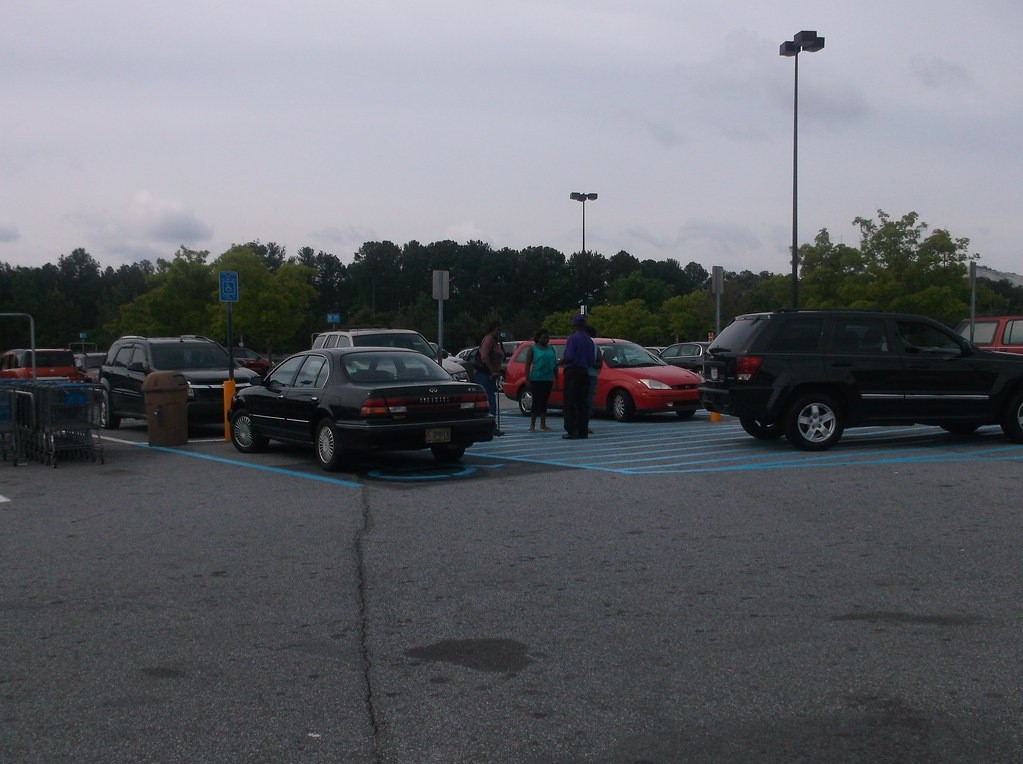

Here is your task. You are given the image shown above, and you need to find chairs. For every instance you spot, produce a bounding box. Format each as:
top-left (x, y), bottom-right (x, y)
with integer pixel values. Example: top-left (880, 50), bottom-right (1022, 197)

top-left (357, 369), bottom-right (389, 380)
top-left (863, 329), bottom-right (882, 351)
top-left (397, 368), bottom-right (425, 378)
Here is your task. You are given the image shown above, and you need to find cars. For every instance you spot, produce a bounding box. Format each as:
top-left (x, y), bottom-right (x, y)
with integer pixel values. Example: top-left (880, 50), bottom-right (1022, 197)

top-left (658, 340), bottom-right (711, 376)
top-left (224, 346), bottom-right (270, 378)
top-left (0, 348), bottom-right (81, 384)
top-left (501, 337), bottom-right (705, 422)
top-left (456, 339), bottom-right (669, 394)
top-left (73, 352), bottom-right (108, 383)
top-left (309, 325), bottom-right (475, 384)
top-left (228, 346), bottom-right (496, 474)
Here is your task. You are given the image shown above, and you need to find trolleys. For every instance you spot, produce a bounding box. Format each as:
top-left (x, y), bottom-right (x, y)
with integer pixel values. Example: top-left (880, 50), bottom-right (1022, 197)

top-left (0, 380), bottom-right (109, 468)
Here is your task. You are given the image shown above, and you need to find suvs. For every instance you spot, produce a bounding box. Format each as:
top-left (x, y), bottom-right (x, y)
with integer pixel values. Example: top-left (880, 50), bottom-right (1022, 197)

top-left (700, 309), bottom-right (1023, 451)
top-left (97, 334), bottom-right (262, 430)
top-left (952, 316), bottom-right (1023, 357)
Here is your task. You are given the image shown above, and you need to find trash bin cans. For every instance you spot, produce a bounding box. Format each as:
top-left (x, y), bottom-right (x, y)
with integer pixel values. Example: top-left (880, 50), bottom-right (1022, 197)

top-left (141, 371), bottom-right (190, 446)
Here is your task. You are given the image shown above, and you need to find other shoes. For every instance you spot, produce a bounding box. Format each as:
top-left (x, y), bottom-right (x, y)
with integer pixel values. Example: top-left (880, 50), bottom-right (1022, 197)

top-left (528, 426), bottom-right (535, 433)
top-left (587, 429), bottom-right (593, 434)
top-left (562, 433), bottom-right (588, 440)
top-left (540, 427), bottom-right (551, 432)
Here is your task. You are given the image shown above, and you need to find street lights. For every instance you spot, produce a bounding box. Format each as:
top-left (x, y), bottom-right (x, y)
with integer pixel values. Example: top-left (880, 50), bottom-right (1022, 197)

top-left (779, 30), bottom-right (827, 304)
top-left (570, 192), bottom-right (599, 317)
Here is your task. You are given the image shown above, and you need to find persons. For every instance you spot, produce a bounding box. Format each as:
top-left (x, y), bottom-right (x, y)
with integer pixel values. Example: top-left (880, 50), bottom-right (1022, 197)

top-left (558, 315), bottom-right (603, 440)
top-left (473, 321), bottom-right (505, 437)
top-left (525, 328), bottom-right (560, 432)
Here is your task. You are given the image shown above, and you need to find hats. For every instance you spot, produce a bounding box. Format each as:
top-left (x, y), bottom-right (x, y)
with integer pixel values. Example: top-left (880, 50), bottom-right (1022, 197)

top-left (573, 314), bottom-right (586, 326)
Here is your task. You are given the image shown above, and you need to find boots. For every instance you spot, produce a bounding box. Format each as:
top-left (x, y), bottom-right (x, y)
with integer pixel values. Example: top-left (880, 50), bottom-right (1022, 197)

top-left (493, 422), bottom-right (505, 436)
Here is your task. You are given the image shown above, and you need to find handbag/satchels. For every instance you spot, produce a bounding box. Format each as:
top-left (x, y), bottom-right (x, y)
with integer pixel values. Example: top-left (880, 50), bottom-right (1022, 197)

top-left (468, 338), bottom-right (490, 369)
